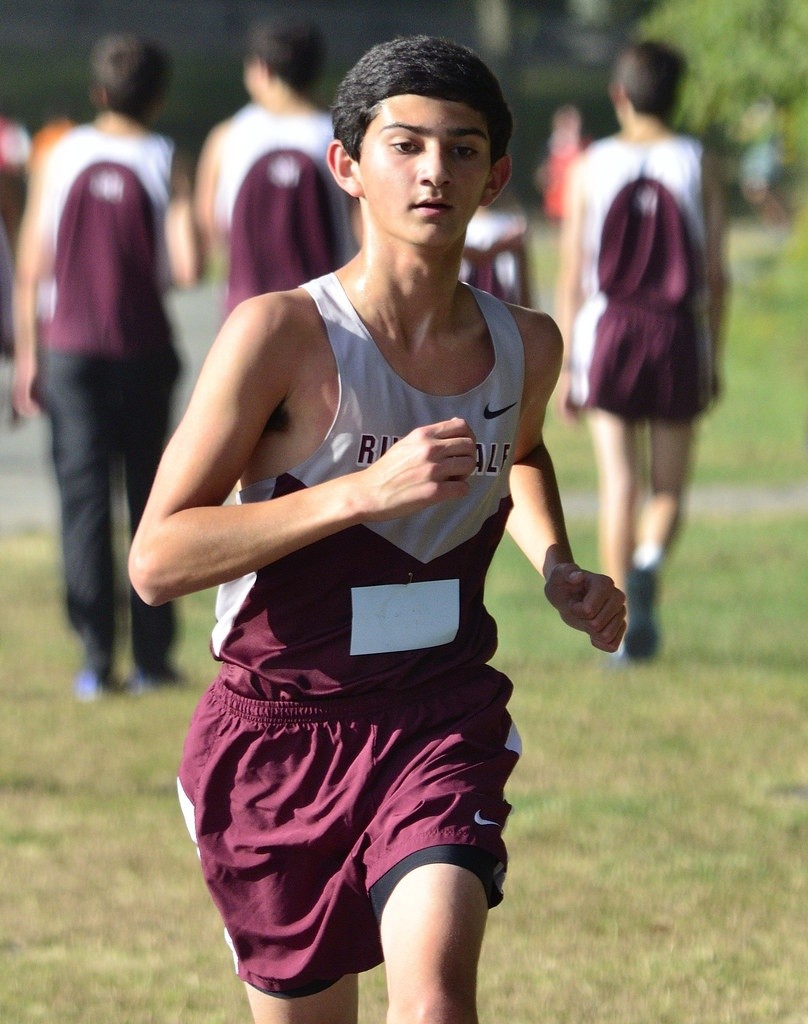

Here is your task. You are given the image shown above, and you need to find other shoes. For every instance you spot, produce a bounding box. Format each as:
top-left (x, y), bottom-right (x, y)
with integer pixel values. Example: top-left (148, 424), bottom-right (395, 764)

top-left (76, 670), bottom-right (107, 696)
top-left (128, 664), bottom-right (177, 693)
top-left (626, 545), bottom-right (657, 662)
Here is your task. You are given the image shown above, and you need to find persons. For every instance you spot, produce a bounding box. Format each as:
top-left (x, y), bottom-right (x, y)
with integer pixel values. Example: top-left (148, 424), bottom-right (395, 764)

top-left (195, 10), bottom-right (792, 327)
top-left (0, 26), bottom-right (210, 701)
top-left (127, 37), bottom-right (661, 1024)
top-left (552, 38), bottom-right (726, 664)
